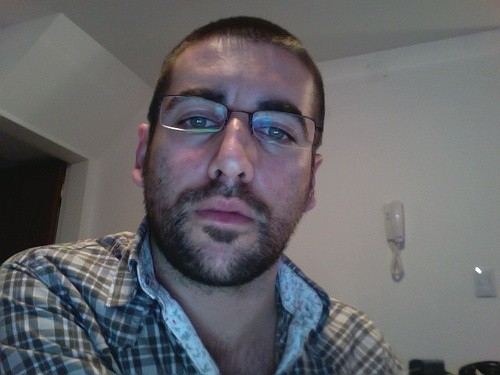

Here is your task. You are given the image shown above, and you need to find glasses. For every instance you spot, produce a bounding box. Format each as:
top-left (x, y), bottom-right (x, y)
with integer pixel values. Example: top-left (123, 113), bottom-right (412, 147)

top-left (150, 95), bottom-right (323, 149)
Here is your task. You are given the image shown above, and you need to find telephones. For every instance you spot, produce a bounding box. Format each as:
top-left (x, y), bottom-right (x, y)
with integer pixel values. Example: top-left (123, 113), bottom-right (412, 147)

top-left (384, 201), bottom-right (404, 246)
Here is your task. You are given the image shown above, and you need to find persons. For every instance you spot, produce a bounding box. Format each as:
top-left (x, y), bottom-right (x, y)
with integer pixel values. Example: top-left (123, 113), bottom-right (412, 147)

top-left (0, 16), bottom-right (405, 375)
